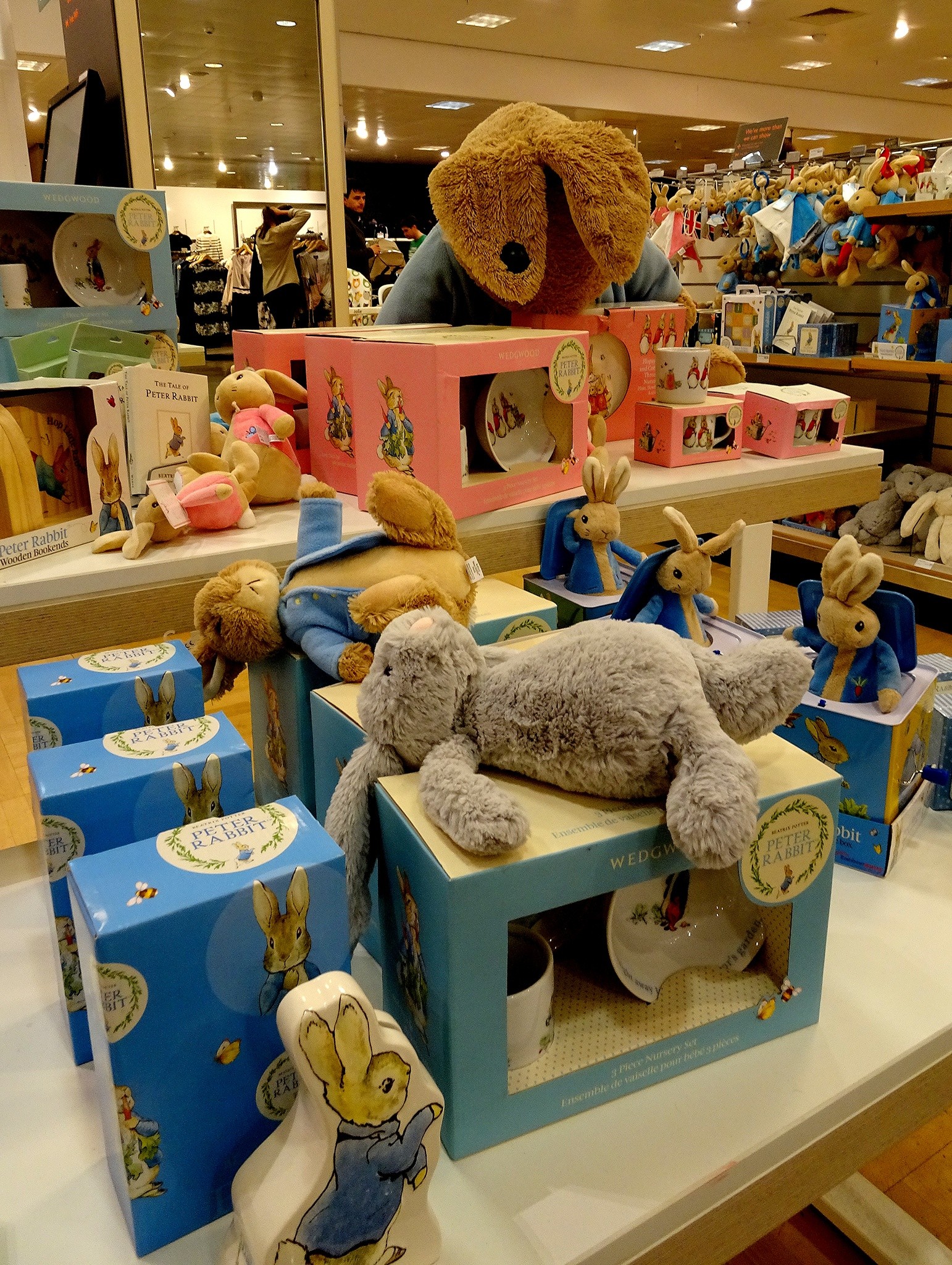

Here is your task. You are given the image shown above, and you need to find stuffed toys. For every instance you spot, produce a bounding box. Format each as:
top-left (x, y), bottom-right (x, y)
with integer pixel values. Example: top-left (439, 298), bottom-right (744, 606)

top-left (782, 537), bottom-right (901, 713)
top-left (660, 143), bottom-right (926, 309)
top-left (189, 472), bottom-right (477, 700)
top-left (374, 102), bottom-right (746, 387)
top-left (324, 599), bottom-right (819, 951)
top-left (563, 457), bottom-right (648, 597)
top-left (901, 260), bottom-right (936, 308)
top-left (91, 367), bottom-right (336, 561)
top-left (633, 504), bottom-right (746, 642)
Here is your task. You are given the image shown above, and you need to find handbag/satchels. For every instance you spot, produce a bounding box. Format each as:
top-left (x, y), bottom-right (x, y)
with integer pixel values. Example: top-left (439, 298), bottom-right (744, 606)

top-left (249, 228), bottom-right (267, 303)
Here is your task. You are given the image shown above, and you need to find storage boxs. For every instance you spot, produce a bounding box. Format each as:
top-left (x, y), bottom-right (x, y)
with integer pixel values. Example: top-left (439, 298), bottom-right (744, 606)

top-left (12, 281), bottom-right (952, 1259)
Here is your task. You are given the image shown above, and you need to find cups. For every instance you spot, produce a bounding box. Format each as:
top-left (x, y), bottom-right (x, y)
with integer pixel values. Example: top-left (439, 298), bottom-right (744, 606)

top-left (460, 424), bottom-right (469, 483)
top-left (683, 414), bottom-right (732, 453)
top-left (0, 264), bottom-right (31, 307)
top-left (915, 171), bottom-right (952, 202)
top-left (506, 922), bottom-right (555, 1072)
top-left (294, 409), bottom-right (309, 449)
top-left (655, 347), bottom-right (711, 404)
top-left (794, 409), bottom-right (822, 445)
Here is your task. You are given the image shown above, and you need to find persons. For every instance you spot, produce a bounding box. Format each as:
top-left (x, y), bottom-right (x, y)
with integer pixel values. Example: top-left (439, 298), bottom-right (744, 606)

top-left (256, 203), bottom-right (311, 327)
top-left (400, 215), bottom-right (427, 258)
top-left (344, 182), bottom-right (381, 277)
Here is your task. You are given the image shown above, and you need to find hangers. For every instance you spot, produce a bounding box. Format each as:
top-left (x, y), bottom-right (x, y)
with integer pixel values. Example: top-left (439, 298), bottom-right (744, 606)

top-left (171, 226), bottom-right (182, 235)
top-left (180, 249), bottom-right (218, 268)
top-left (202, 225), bottom-right (212, 235)
top-left (235, 232), bottom-right (329, 259)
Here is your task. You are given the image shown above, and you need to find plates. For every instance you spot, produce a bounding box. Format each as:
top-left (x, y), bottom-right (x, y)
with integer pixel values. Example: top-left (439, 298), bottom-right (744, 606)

top-left (931, 146), bottom-right (952, 188)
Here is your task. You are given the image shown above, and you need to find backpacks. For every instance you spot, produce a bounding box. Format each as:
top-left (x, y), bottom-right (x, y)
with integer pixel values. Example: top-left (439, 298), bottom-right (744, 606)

top-left (370, 240), bottom-right (406, 278)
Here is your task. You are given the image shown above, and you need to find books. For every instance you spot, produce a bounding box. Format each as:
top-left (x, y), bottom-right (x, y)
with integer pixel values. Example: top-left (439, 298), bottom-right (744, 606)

top-left (122, 366), bottom-right (212, 497)
top-left (772, 301), bottom-right (834, 355)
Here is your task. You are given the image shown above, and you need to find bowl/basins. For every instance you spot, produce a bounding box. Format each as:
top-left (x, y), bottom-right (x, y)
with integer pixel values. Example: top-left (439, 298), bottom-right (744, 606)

top-left (606, 861), bottom-right (768, 1004)
top-left (52, 213), bottom-right (146, 307)
top-left (474, 368), bottom-right (556, 471)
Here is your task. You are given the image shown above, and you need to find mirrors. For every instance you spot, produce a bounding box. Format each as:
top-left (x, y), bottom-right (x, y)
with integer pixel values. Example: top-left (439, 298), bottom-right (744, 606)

top-left (136, 0), bottom-right (336, 358)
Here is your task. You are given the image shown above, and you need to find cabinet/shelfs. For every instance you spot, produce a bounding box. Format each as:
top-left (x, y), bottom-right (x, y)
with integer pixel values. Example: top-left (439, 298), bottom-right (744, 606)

top-left (707, 196), bottom-right (952, 603)
